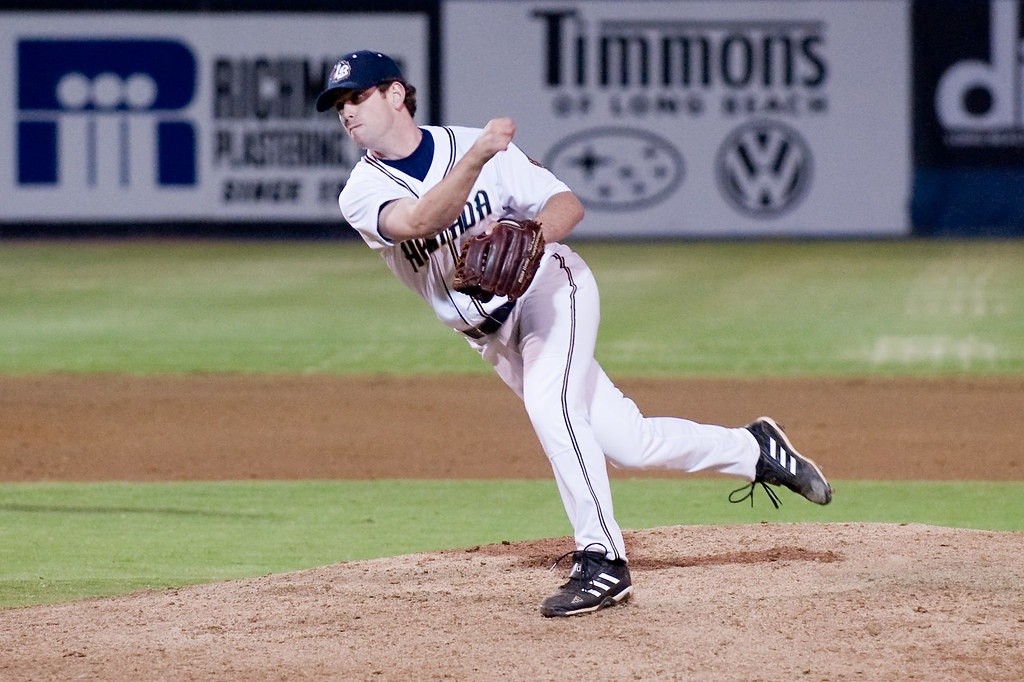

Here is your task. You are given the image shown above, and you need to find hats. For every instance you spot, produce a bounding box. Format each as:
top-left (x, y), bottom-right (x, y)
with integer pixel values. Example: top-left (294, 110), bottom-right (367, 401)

top-left (314, 49), bottom-right (403, 112)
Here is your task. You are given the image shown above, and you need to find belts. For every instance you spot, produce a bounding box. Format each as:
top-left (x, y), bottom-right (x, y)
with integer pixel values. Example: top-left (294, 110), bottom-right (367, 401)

top-left (462, 297), bottom-right (517, 340)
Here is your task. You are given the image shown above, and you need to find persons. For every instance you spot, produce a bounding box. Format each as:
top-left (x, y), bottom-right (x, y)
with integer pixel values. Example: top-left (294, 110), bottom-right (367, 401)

top-left (314, 48), bottom-right (834, 619)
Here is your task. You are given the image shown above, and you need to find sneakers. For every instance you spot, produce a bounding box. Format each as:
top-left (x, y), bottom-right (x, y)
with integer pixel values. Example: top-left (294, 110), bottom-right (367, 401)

top-left (539, 543), bottom-right (633, 617)
top-left (729, 416), bottom-right (831, 509)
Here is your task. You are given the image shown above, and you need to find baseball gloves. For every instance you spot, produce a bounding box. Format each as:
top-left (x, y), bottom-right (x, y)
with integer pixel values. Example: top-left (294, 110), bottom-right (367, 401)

top-left (453, 217), bottom-right (546, 303)
